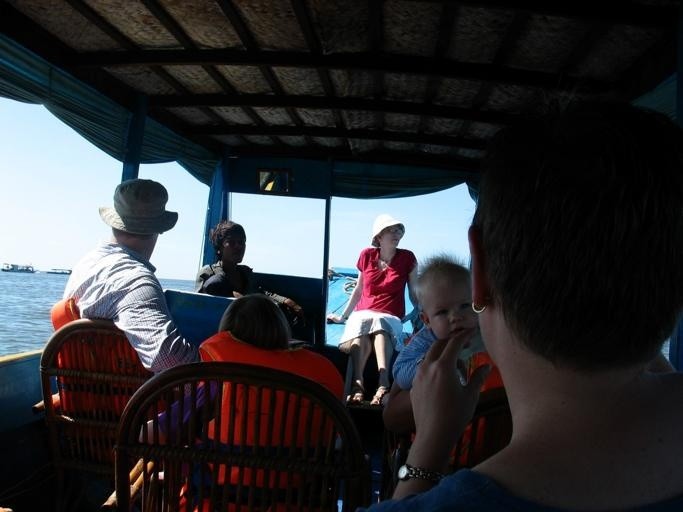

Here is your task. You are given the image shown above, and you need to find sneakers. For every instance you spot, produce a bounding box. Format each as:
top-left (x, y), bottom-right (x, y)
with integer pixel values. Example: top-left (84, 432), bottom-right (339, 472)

top-left (370, 387), bottom-right (388, 404)
top-left (347, 390), bottom-right (365, 403)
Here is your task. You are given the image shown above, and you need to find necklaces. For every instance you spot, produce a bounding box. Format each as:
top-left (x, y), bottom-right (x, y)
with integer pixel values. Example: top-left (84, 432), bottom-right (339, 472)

top-left (377, 256), bottom-right (392, 266)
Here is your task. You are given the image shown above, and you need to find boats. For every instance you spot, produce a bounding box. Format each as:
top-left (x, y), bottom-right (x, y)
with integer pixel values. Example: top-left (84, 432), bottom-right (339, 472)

top-left (46, 269), bottom-right (72, 274)
top-left (2, 263), bottom-right (37, 273)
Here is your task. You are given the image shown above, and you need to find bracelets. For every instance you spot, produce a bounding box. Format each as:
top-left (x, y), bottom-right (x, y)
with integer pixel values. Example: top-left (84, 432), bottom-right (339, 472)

top-left (341, 314), bottom-right (349, 320)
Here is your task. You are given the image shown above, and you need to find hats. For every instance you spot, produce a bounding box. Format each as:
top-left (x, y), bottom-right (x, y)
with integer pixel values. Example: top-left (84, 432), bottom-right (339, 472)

top-left (372, 213), bottom-right (405, 247)
top-left (99, 180), bottom-right (177, 235)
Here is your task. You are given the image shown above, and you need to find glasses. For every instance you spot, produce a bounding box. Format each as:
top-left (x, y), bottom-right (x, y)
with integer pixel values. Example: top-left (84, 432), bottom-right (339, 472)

top-left (389, 228), bottom-right (403, 234)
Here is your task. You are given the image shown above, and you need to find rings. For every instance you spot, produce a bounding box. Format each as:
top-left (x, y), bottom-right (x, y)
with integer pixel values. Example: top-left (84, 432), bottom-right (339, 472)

top-left (416, 356), bottom-right (425, 363)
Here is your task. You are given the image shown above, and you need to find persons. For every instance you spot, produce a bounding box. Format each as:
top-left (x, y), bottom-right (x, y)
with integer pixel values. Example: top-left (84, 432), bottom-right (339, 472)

top-left (326, 213), bottom-right (419, 405)
top-left (137, 291), bottom-right (343, 512)
top-left (392, 256), bottom-right (494, 391)
top-left (355, 102), bottom-right (683, 512)
top-left (383, 306), bottom-right (504, 468)
top-left (194, 220), bottom-right (307, 342)
top-left (61, 178), bottom-right (202, 395)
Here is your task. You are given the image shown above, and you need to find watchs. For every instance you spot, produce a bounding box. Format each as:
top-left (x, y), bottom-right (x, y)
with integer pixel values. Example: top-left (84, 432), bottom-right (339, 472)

top-left (397, 462), bottom-right (445, 482)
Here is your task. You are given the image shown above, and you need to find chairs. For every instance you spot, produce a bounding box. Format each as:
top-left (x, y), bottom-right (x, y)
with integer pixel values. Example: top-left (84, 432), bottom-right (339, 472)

top-left (379, 386), bottom-right (512, 505)
top-left (39, 317), bottom-right (154, 512)
top-left (102, 360), bottom-right (373, 509)
